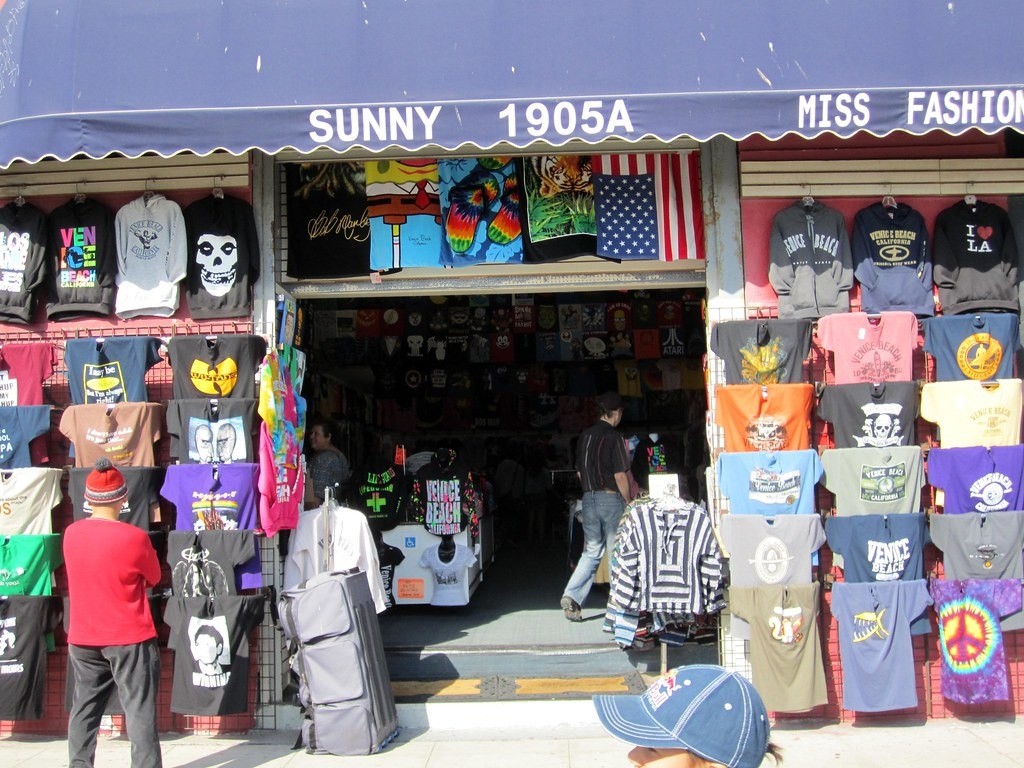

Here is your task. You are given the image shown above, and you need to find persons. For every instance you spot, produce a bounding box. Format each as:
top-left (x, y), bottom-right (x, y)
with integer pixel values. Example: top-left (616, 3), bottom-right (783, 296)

top-left (63, 458), bottom-right (162, 768)
top-left (560, 391), bottom-right (636, 622)
top-left (306, 419), bottom-right (351, 510)
top-left (592, 663), bottom-right (786, 768)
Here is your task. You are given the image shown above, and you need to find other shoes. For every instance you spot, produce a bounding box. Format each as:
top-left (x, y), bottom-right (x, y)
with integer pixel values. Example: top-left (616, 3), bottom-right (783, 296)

top-left (508, 538), bottom-right (519, 547)
top-left (561, 597), bottom-right (582, 621)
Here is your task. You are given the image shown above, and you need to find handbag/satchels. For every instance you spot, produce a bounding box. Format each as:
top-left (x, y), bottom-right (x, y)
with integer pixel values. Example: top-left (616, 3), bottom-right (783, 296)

top-left (502, 491), bottom-right (512, 511)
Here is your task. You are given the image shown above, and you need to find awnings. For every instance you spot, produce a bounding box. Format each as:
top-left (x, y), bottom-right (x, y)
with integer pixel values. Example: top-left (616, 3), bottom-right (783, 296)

top-left (0, 1), bottom-right (1023, 170)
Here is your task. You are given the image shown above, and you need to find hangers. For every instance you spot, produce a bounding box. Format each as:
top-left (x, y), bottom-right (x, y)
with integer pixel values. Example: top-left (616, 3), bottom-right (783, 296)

top-left (143, 179), bottom-right (156, 205)
top-left (882, 181), bottom-right (899, 209)
top-left (965, 180), bottom-right (979, 206)
top-left (324, 486), bottom-right (339, 511)
top-left (212, 175), bottom-right (225, 199)
top-left (802, 184), bottom-right (815, 208)
top-left (14, 185), bottom-right (25, 208)
top-left (74, 180), bottom-right (86, 204)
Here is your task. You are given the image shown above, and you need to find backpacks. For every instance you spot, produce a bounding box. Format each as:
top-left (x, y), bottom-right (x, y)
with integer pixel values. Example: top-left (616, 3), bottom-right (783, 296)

top-left (529, 442), bottom-right (566, 493)
top-left (278, 566), bottom-right (398, 756)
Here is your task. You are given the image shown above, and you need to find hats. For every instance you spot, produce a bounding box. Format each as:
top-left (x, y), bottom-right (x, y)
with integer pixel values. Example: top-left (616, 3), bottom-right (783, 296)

top-left (84, 458), bottom-right (129, 503)
top-left (593, 665), bottom-right (769, 768)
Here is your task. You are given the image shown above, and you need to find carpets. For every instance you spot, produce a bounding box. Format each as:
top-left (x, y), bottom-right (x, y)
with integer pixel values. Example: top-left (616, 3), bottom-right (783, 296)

top-left (385, 649), bottom-right (647, 704)
